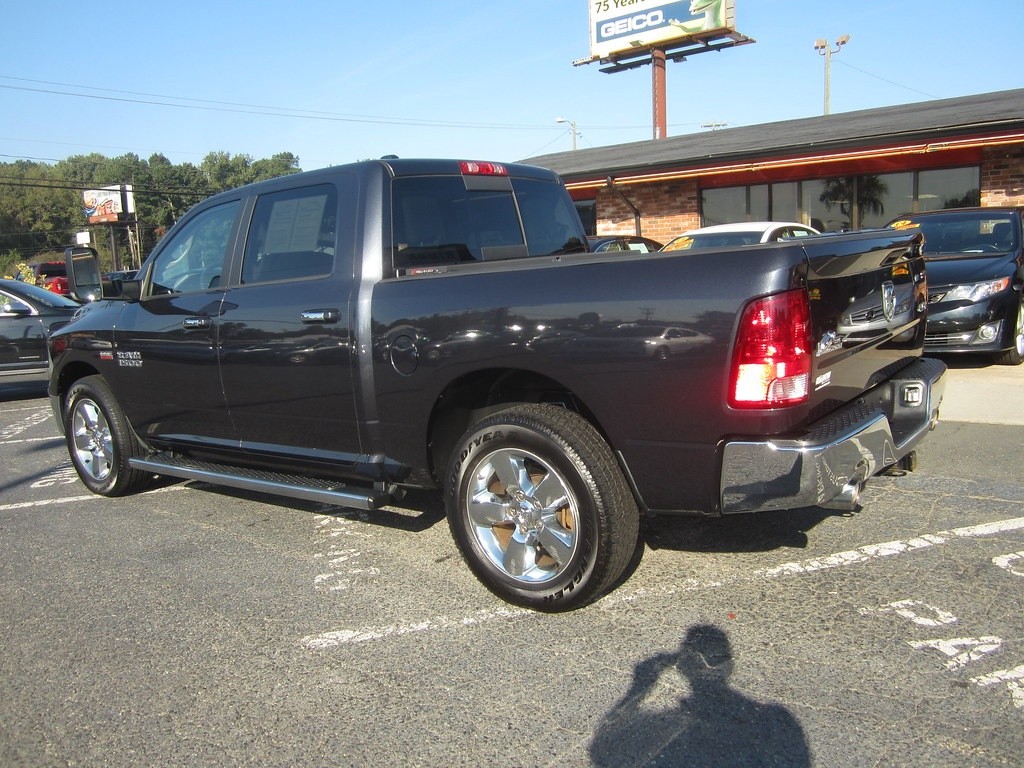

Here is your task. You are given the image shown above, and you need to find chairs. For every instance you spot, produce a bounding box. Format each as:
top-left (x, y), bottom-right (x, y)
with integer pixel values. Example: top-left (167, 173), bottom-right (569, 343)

top-left (987, 223), bottom-right (1014, 252)
top-left (941, 232), bottom-right (966, 252)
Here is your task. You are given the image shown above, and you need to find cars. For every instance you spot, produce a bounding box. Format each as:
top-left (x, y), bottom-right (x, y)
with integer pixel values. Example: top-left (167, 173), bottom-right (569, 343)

top-left (584, 235), bottom-right (661, 256)
top-left (102, 270), bottom-right (139, 291)
top-left (0, 277), bottom-right (86, 392)
top-left (655, 220), bottom-right (823, 255)
top-left (878, 206), bottom-right (1023, 366)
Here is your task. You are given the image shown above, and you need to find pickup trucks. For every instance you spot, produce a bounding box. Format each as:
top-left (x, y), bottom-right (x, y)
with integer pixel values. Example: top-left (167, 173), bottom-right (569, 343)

top-left (42, 154), bottom-right (953, 614)
top-left (12, 262), bottom-right (110, 297)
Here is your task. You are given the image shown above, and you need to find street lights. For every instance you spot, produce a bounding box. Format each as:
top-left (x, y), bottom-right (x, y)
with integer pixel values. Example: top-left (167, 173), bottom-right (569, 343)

top-left (815, 34), bottom-right (851, 115)
top-left (557, 117), bottom-right (576, 152)
